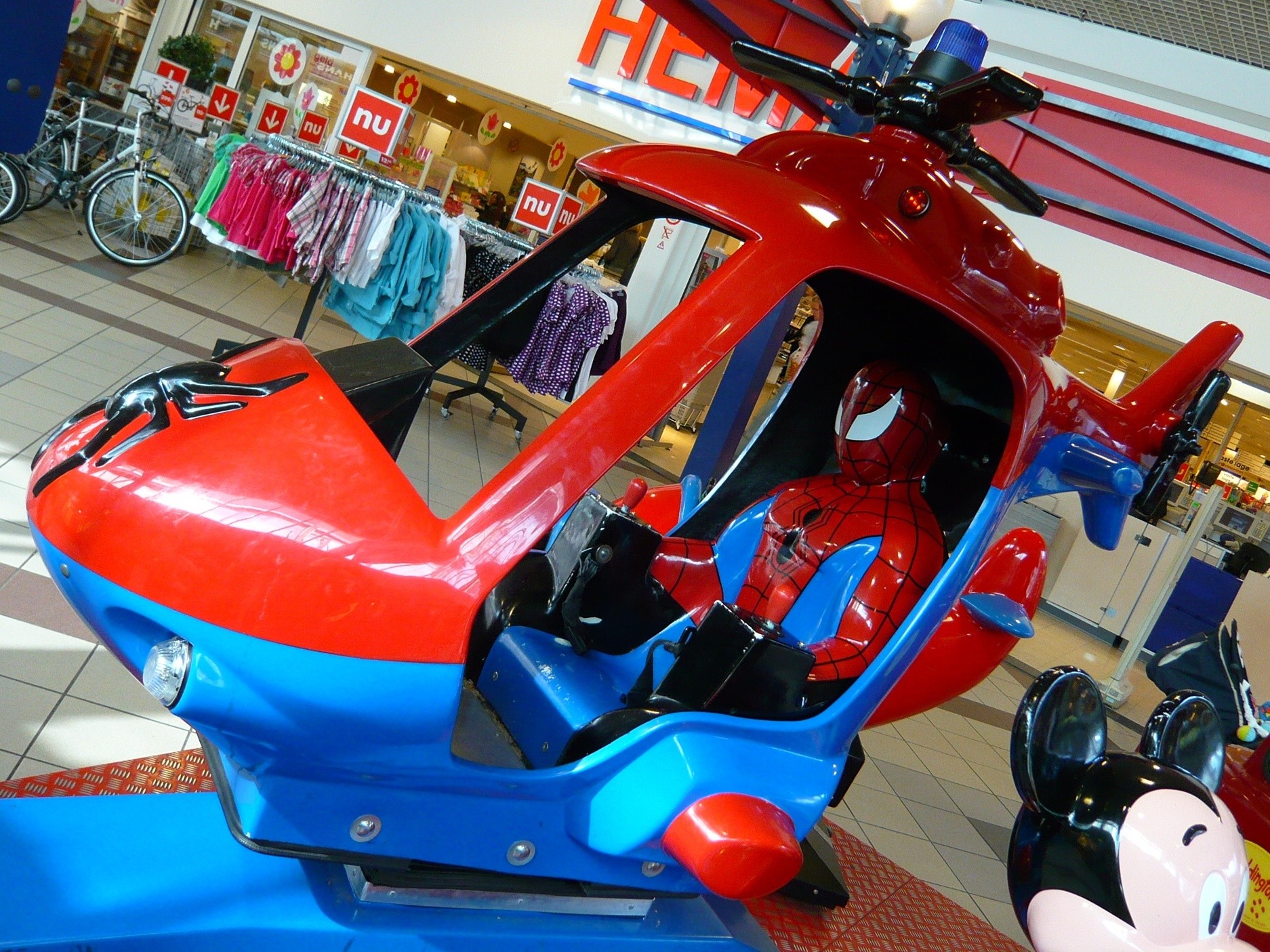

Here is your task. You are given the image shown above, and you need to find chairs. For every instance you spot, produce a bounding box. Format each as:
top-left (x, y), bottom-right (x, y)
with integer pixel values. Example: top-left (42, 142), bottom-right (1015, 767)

top-left (478, 617), bottom-right (698, 768)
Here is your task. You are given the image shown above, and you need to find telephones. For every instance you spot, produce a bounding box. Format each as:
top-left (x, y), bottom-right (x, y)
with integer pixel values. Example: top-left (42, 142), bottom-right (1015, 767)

top-left (1194, 460), bottom-right (1222, 489)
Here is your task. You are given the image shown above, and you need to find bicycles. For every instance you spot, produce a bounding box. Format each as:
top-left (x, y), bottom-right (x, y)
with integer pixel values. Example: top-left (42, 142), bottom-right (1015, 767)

top-left (0, 81), bottom-right (192, 267)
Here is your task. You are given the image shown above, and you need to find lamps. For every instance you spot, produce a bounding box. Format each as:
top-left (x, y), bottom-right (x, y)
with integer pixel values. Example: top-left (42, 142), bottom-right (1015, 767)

top-left (385, 65), bottom-right (511, 130)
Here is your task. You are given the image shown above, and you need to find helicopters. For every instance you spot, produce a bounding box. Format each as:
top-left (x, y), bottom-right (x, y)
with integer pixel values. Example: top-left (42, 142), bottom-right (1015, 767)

top-left (5, 0), bottom-right (1244, 952)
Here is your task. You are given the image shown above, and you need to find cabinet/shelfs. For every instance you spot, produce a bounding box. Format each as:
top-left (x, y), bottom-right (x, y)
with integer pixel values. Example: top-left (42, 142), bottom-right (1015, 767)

top-left (56, 21), bottom-right (142, 110)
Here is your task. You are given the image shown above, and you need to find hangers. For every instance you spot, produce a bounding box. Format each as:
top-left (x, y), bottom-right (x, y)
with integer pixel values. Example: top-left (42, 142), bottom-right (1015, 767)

top-left (266, 133), bottom-right (444, 216)
top-left (464, 216), bottom-right (603, 281)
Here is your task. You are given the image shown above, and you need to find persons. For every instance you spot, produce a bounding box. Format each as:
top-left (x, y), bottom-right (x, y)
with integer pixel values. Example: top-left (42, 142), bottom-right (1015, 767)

top-left (498, 201), bottom-right (516, 230)
top-left (604, 223), bottom-right (643, 282)
top-left (475, 191), bottom-right (506, 226)
top-left (618, 236), bottom-right (647, 287)
